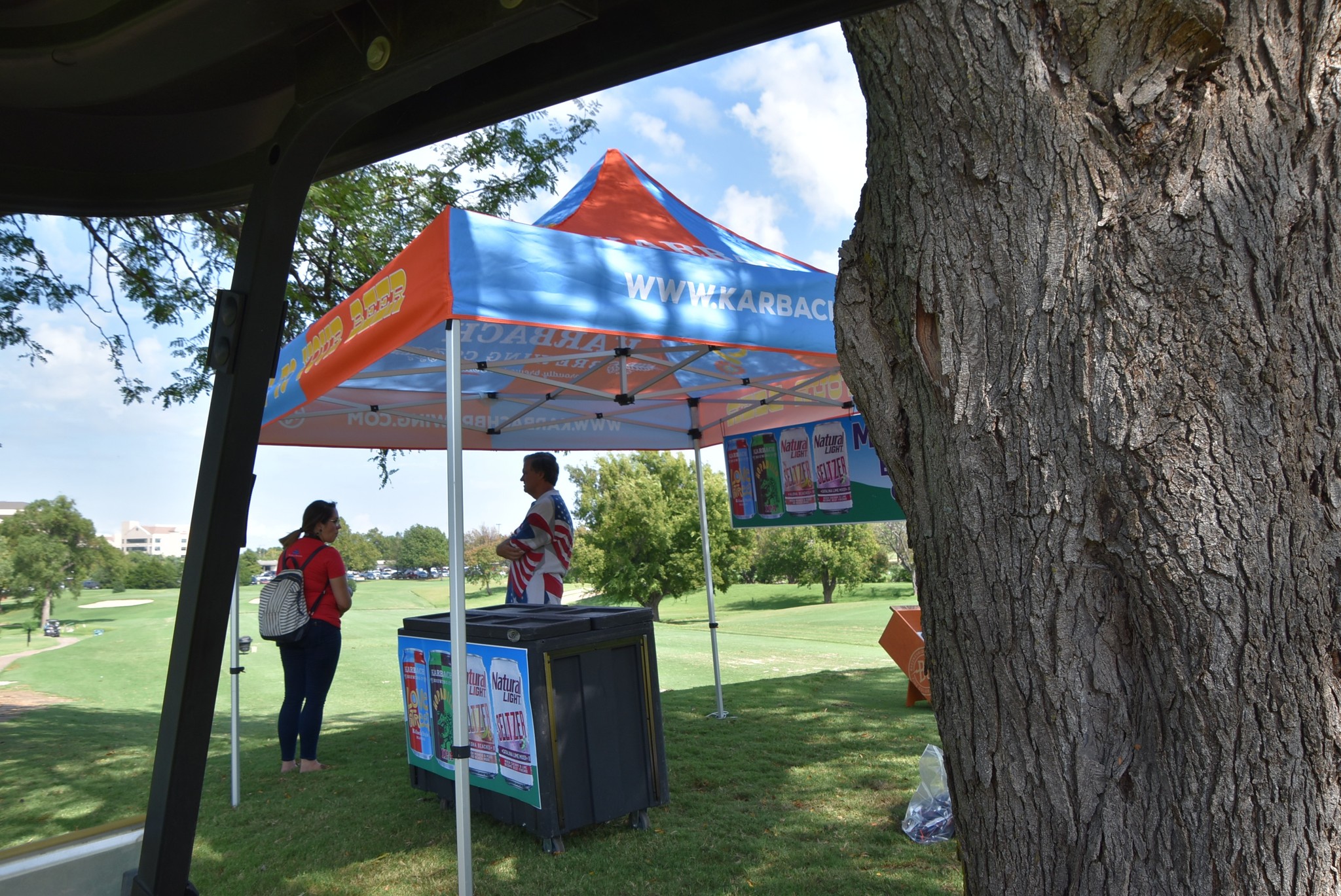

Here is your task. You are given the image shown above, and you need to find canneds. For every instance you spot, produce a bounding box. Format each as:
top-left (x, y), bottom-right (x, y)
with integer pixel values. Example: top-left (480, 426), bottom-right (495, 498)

top-left (428, 650), bottom-right (456, 771)
top-left (401, 647), bottom-right (434, 760)
top-left (780, 427), bottom-right (817, 517)
top-left (465, 653), bottom-right (499, 779)
top-left (751, 431), bottom-right (785, 519)
top-left (812, 421), bottom-right (853, 514)
top-left (490, 656), bottom-right (534, 791)
top-left (726, 437), bottom-right (757, 519)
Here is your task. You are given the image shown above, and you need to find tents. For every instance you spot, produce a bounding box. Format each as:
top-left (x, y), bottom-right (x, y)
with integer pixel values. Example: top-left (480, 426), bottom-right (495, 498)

top-left (231, 148), bottom-right (905, 896)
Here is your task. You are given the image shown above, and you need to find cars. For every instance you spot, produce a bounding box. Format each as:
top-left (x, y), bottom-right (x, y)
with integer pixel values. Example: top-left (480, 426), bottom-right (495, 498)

top-left (83, 580), bottom-right (101, 590)
top-left (346, 565), bottom-right (480, 582)
top-left (253, 571), bottom-right (278, 584)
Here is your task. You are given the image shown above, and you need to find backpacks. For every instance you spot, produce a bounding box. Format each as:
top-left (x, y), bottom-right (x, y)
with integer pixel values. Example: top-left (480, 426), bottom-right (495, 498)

top-left (258, 545), bottom-right (333, 643)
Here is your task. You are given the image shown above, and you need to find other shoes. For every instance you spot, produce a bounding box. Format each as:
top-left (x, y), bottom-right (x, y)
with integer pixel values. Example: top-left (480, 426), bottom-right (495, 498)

top-left (319, 764), bottom-right (334, 772)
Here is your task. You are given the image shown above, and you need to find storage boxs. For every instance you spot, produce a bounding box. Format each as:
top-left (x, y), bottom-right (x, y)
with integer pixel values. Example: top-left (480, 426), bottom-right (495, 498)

top-left (398, 604), bottom-right (670, 852)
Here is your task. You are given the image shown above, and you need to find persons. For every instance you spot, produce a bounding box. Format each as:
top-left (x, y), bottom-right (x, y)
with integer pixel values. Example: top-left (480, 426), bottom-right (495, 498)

top-left (496, 452), bottom-right (574, 606)
top-left (277, 500), bottom-right (353, 775)
